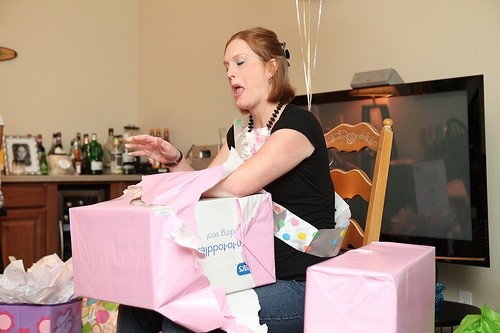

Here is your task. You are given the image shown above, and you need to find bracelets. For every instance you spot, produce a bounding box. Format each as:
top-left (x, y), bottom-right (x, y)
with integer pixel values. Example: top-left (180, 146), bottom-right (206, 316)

top-left (163, 147), bottom-right (183, 167)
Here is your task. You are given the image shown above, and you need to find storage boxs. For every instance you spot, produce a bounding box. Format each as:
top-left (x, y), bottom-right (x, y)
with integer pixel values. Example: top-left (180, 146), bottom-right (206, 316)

top-left (304, 242), bottom-right (436, 332)
top-left (0, 194), bottom-right (436, 333)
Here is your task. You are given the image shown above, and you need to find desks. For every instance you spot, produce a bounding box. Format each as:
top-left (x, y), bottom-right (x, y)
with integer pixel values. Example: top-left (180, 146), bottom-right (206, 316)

top-left (436, 300), bottom-right (481, 333)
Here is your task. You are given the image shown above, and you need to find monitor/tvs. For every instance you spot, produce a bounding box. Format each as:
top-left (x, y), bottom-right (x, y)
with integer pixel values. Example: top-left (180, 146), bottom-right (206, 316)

top-left (289, 74), bottom-right (490, 268)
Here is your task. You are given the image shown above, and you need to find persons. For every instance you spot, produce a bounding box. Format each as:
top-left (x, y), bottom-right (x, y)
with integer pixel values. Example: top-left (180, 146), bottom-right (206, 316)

top-left (12, 144), bottom-right (30, 166)
top-left (115, 25), bottom-right (337, 333)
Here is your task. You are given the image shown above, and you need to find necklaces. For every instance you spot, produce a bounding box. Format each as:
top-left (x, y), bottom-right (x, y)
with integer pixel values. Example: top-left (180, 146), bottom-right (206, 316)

top-left (247, 98), bottom-right (285, 133)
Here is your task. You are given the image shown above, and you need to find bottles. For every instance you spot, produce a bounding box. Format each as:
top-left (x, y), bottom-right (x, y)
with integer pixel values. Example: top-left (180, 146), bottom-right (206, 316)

top-left (156, 129), bottom-right (161, 173)
top-left (54, 132), bottom-right (66, 154)
top-left (36, 134), bottom-right (49, 174)
top-left (146, 129), bottom-right (155, 173)
top-left (70, 126), bottom-right (141, 174)
top-left (164, 128), bottom-right (170, 141)
top-left (47, 134), bottom-right (57, 154)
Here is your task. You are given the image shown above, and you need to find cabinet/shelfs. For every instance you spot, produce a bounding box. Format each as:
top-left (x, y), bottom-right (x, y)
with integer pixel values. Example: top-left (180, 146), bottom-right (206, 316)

top-left (0, 175), bottom-right (150, 279)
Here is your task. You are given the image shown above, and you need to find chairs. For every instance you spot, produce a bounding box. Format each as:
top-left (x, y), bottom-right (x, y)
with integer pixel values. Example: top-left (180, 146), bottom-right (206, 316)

top-left (321, 117), bottom-right (393, 249)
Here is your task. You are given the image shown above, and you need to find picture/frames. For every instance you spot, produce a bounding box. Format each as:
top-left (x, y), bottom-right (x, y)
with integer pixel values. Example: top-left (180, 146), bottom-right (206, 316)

top-left (3, 134), bottom-right (42, 175)
